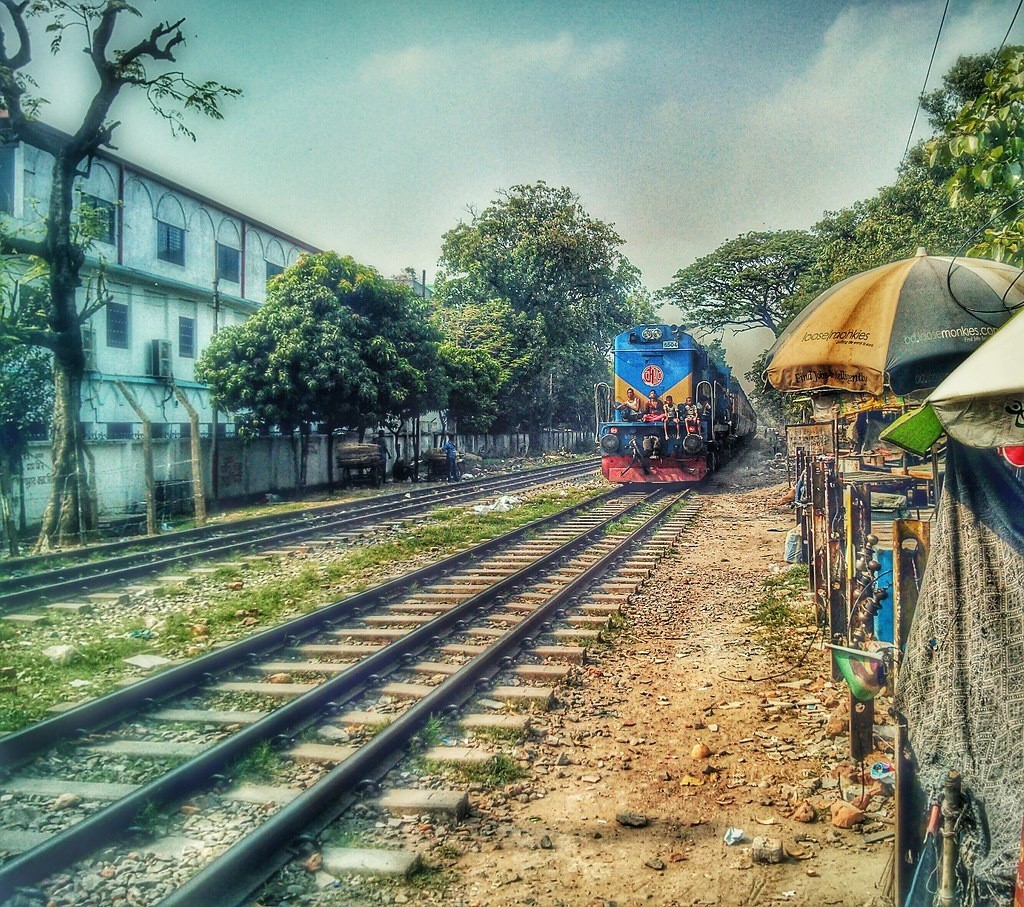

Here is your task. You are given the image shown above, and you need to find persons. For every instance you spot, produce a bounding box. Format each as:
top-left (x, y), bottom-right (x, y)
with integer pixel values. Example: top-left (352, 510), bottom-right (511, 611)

top-left (685, 396), bottom-right (711, 440)
top-left (438, 435), bottom-right (459, 482)
top-left (610, 388), bottom-right (680, 440)
top-left (367, 430), bottom-right (392, 484)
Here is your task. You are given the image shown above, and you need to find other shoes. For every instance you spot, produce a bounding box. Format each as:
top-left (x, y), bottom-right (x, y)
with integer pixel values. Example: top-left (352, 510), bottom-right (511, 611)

top-left (665, 435), bottom-right (670, 441)
top-left (688, 433), bottom-right (690, 436)
top-left (448, 479), bottom-right (456, 483)
top-left (453, 477), bottom-right (459, 482)
top-left (383, 480), bottom-right (390, 484)
top-left (676, 435), bottom-right (680, 439)
top-left (700, 435), bottom-right (703, 440)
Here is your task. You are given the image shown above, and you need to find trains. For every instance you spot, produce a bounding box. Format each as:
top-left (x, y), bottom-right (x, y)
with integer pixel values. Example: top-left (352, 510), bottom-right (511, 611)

top-left (594, 322), bottom-right (759, 483)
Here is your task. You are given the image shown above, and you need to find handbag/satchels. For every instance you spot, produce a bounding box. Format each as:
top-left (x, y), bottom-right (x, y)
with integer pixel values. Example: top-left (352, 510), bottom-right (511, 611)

top-left (783, 523), bottom-right (803, 564)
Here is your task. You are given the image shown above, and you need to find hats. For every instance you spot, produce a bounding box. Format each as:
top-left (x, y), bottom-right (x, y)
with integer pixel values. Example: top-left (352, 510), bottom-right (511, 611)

top-left (398, 457), bottom-right (406, 462)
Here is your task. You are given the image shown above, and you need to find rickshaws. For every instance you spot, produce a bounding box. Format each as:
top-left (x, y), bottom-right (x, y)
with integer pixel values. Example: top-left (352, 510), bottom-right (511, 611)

top-left (427, 458), bottom-right (467, 482)
top-left (342, 457), bottom-right (390, 490)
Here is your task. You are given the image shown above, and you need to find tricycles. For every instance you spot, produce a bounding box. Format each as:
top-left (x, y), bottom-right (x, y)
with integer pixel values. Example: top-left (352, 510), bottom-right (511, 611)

top-left (765, 246), bottom-right (1024, 905)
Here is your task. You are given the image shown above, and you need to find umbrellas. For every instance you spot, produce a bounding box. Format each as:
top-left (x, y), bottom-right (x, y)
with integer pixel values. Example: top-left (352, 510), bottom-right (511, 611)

top-left (760, 246), bottom-right (1024, 467)
